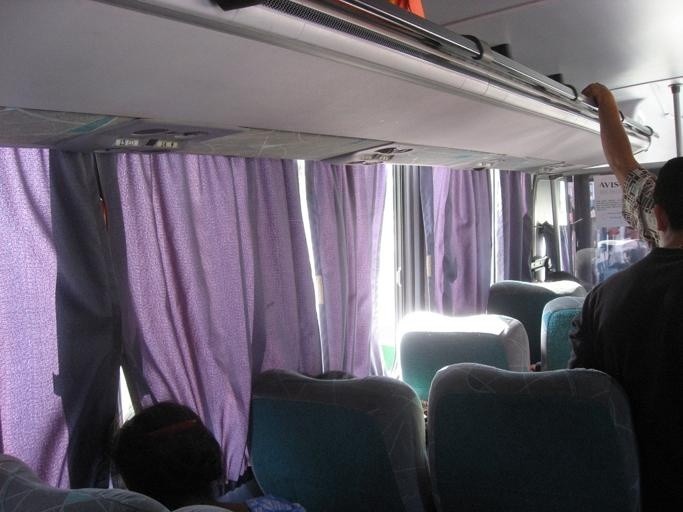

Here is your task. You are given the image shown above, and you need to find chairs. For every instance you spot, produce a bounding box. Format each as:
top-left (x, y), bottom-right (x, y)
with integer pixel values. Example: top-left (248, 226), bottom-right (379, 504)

top-left (0, 276), bottom-right (643, 512)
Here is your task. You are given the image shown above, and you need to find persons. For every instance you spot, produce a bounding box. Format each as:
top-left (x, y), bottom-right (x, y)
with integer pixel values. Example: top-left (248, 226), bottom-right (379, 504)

top-left (580, 82), bottom-right (672, 256)
top-left (112, 401), bottom-right (307, 512)
top-left (313, 372), bottom-right (428, 450)
top-left (544, 272), bottom-right (579, 283)
top-left (568, 156), bottom-right (683, 510)
